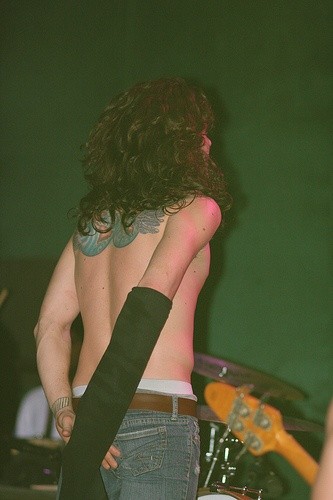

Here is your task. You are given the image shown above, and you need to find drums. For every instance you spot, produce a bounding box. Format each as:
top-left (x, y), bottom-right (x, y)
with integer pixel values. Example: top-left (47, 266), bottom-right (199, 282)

top-left (196, 486), bottom-right (254, 500)
top-left (6, 437), bottom-right (62, 489)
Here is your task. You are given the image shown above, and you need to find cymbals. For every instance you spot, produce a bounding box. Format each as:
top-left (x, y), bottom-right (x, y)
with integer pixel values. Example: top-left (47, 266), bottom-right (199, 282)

top-left (194, 405), bottom-right (326, 433)
top-left (193, 352), bottom-right (307, 401)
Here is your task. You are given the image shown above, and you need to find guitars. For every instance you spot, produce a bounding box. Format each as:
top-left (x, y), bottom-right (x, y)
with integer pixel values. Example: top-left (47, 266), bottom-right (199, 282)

top-left (205, 379), bottom-right (321, 485)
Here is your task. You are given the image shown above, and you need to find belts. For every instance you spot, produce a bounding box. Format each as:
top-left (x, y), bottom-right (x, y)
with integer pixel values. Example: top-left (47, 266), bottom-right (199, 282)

top-left (71, 392), bottom-right (197, 418)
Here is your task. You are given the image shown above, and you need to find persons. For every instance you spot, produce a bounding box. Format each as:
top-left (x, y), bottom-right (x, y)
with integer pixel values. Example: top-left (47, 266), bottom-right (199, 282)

top-left (33, 77), bottom-right (222, 500)
top-left (311, 400), bottom-right (333, 500)
top-left (12, 386), bottom-right (68, 450)
